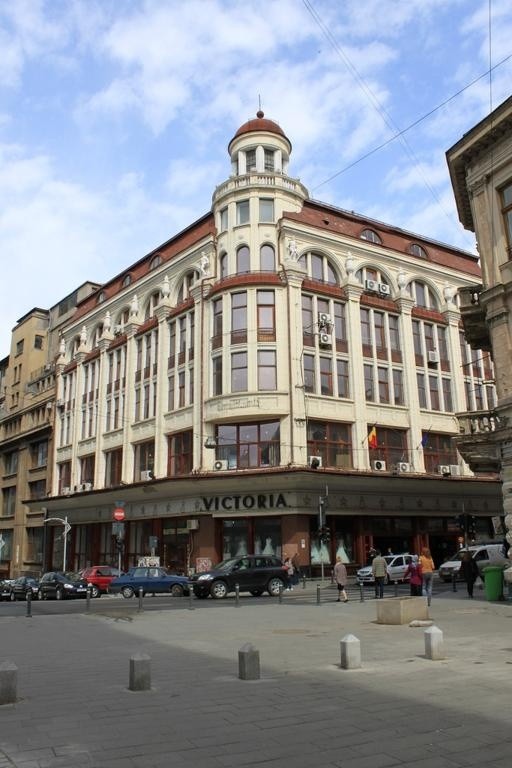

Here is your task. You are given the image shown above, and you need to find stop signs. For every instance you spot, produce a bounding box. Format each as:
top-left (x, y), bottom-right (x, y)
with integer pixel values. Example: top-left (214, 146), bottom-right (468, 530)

top-left (114, 509), bottom-right (126, 521)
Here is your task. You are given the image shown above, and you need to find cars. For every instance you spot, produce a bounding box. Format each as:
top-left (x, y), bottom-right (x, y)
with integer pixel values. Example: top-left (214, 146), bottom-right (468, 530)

top-left (1, 566), bottom-right (126, 601)
top-left (358, 553), bottom-right (423, 585)
top-left (112, 566), bottom-right (192, 598)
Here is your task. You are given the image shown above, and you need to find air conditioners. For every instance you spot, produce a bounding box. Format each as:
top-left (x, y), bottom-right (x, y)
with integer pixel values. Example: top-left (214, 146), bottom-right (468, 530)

top-left (379, 284), bottom-right (390, 296)
top-left (365, 280), bottom-right (378, 292)
top-left (427, 351), bottom-right (439, 363)
top-left (114, 325), bottom-right (121, 335)
top-left (319, 312), bottom-right (331, 322)
top-left (437, 464), bottom-right (449, 476)
top-left (397, 463), bottom-right (410, 473)
top-left (319, 333), bottom-right (332, 345)
top-left (213, 460), bottom-right (228, 471)
top-left (140, 471), bottom-right (152, 481)
top-left (82, 483), bottom-right (91, 491)
top-left (373, 461), bottom-right (386, 471)
top-left (187, 520), bottom-right (200, 530)
top-left (449, 465), bottom-right (460, 476)
top-left (74, 485), bottom-right (82, 493)
top-left (44, 364), bottom-right (50, 372)
top-left (309, 456), bottom-right (322, 468)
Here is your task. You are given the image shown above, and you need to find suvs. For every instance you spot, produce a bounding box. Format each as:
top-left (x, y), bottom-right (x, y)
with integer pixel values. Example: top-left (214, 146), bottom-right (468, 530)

top-left (192, 554), bottom-right (290, 599)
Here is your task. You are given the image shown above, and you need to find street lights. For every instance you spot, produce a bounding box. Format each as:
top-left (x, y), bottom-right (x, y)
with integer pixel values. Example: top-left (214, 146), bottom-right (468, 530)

top-left (43, 516), bottom-right (71, 571)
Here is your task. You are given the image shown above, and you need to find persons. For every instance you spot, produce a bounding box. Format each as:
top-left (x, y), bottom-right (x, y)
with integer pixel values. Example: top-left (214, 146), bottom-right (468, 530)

top-left (458, 551), bottom-right (479, 600)
top-left (283, 552), bottom-right (300, 592)
top-left (334, 556), bottom-right (349, 603)
top-left (455, 536), bottom-right (465, 552)
top-left (401, 554), bottom-right (423, 597)
top-left (419, 548), bottom-right (434, 605)
top-left (371, 549), bottom-right (388, 599)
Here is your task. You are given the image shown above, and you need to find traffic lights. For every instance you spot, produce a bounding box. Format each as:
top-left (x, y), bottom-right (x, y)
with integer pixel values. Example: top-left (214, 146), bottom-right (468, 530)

top-left (468, 514), bottom-right (475, 533)
top-left (454, 512), bottom-right (464, 531)
top-left (320, 525), bottom-right (331, 544)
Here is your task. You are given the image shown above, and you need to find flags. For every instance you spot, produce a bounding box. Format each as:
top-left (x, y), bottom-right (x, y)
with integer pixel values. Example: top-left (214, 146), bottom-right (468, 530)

top-left (421, 432), bottom-right (428, 448)
top-left (369, 426), bottom-right (378, 449)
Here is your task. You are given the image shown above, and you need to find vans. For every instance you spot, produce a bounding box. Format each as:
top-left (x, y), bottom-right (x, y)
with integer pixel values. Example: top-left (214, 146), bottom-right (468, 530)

top-left (440, 544), bottom-right (509, 582)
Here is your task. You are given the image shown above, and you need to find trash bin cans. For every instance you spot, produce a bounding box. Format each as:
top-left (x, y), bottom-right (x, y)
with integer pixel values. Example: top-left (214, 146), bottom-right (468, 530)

top-left (481, 563), bottom-right (504, 602)
